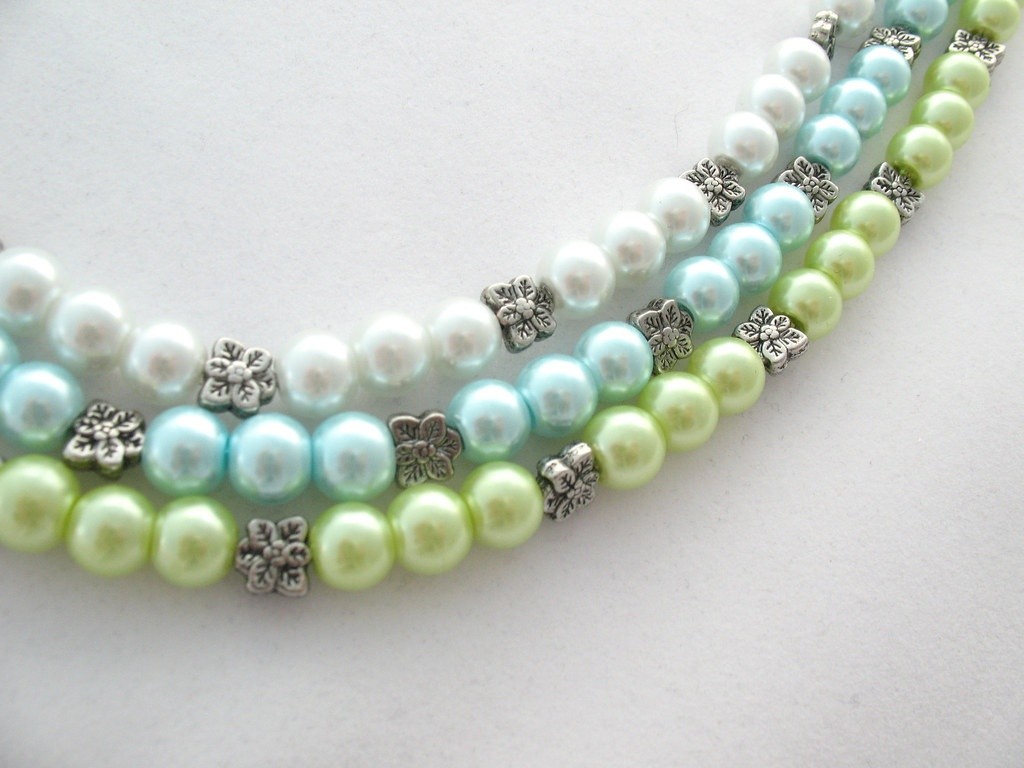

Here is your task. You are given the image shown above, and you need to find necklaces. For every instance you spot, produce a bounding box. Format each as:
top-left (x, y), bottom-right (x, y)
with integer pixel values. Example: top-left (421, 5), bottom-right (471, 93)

top-left (0, 0), bottom-right (1021, 597)
